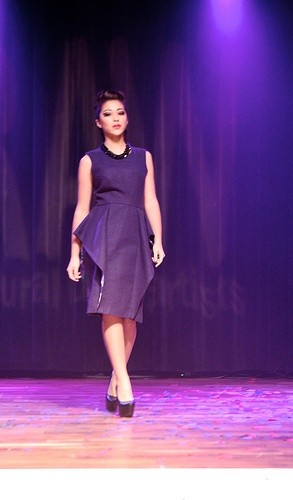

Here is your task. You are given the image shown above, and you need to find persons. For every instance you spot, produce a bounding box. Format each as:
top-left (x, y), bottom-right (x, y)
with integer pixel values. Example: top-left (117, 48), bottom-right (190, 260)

top-left (65, 88), bottom-right (167, 418)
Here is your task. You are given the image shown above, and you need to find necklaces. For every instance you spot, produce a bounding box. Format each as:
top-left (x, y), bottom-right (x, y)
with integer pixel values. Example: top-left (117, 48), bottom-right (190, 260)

top-left (100, 142), bottom-right (132, 160)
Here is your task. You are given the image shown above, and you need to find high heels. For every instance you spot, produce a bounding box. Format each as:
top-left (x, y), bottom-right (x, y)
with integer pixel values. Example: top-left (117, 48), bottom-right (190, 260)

top-left (106, 376), bottom-right (117, 413)
top-left (118, 400), bottom-right (135, 417)
